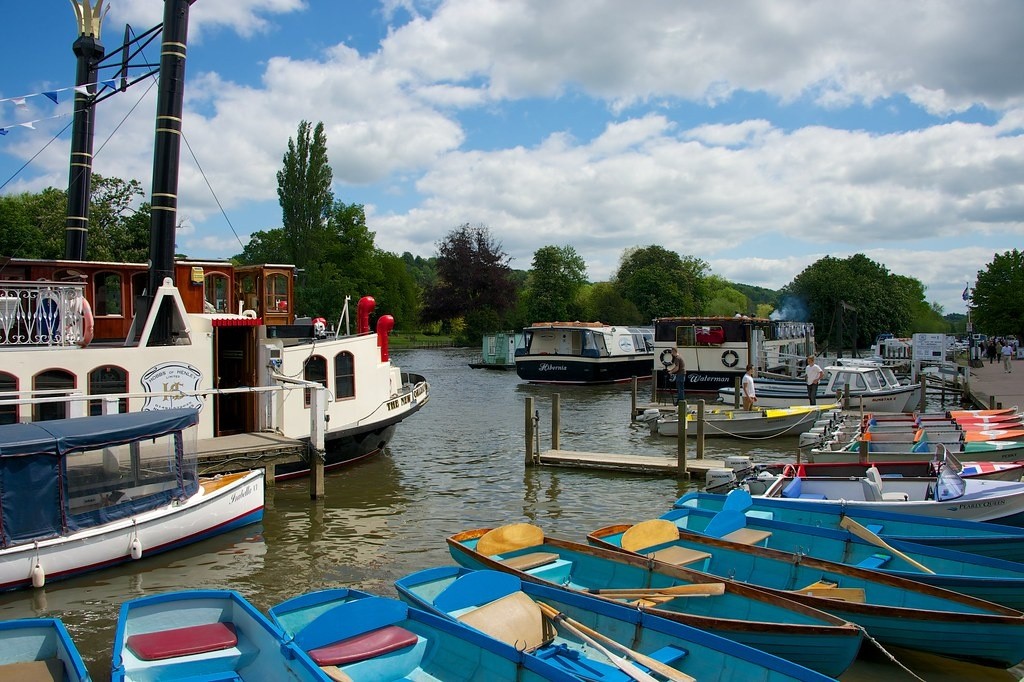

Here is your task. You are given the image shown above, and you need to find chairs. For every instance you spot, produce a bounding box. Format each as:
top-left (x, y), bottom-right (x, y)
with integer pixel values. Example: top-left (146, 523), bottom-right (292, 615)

top-left (862, 478), bottom-right (883, 502)
top-left (865, 467), bottom-right (910, 501)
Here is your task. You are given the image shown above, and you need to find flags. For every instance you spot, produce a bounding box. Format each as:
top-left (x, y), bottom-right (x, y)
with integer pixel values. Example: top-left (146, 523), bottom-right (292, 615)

top-left (962, 282), bottom-right (969, 301)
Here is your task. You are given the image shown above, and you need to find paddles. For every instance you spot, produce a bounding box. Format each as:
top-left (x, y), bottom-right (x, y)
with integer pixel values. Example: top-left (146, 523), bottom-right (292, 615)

top-left (536, 600), bottom-right (697, 682)
top-left (599, 593), bottom-right (711, 598)
top-left (840, 515), bottom-right (935, 575)
top-left (785, 588), bottom-right (866, 603)
top-left (534, 603), bottom-right (659, 682)
top-left (583, 582), bottom-right (726, 595)
top-left (320, 665), bottom-right (353, 682)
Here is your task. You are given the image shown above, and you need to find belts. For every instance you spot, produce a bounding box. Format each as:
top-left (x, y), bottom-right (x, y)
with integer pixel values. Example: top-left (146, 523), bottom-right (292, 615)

top-left (1004, 355), bottom-right (1010, 356)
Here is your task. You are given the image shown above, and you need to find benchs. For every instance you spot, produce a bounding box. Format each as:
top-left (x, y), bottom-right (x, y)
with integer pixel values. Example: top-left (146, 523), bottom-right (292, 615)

top-left (781, 476), bottom-right (828, 500)
top-left (310, 625), bottom-right (435, 682)
top-left (744, 510), bottom-right (773, 521)
top-left (498, 551), bottom-right (578, 586)
top-left (857, 553), bottom-right (893, 569)
top-left (122, 621), bottom-right (260, 682)
top-left (721, 527), bottom-right (773, 548)
top-left (0, 657), bottom-right (69, 682)
top-left (866, 524), bottom-right (884, 535)
top-left (801, 580), bottom-right (838, 588)
top-left (645, 545), bottom-right (714, 572)
top-left (599, 643), bottom-right (689, 682)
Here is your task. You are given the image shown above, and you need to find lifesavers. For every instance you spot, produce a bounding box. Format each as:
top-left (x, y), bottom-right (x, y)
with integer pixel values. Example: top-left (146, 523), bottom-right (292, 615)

top-left (312, 318), bottom-right (327, 327)
top-left (603, 325), bottom-right (609, 327)
top-left (898, 338), bottom-right (911, 341)
top-left (67, 298), bottom-right (95, 348)
top-left (660, 349), bottom-right (675, 366)
top-left (721, 350), bottom-right (739, 367)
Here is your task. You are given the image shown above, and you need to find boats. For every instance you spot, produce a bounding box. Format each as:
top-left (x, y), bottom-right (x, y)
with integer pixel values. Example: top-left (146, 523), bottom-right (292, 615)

top-left (466, 328), bottom-right (530, 369)
top-left (0, 0), bottom-right (435, 486)
top-left (393, 564), bottom-right (840, 682)
top-left (585, 332), bottom-right (1024, 671)
top-left (513, 320), bottom-right (656, 387)
top-left (0, 616), bottom-right (94, 682)
top-left (106, 589), bottom-right (333, 682)
top-left (267, 588), bottom-right (586, 682)
top-left (650, 312), bottom-right (817, 396)
top-left (444, 522), bottom-right (865, 679)
top-left (0, 404), bottom-right (267, 594)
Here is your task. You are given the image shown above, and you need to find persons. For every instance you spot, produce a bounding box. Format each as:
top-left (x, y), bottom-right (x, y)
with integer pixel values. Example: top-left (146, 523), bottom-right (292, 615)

top-left (733, 310), bottom-right (748, 318)
top-left (667, 347), bottom-right (686, 406)
top-left (987, 337), bottom-right (1014, 373)
top-left (742, 364), bottom-right (757, 411)
top-left (806, 356), bottom-right (824, 405)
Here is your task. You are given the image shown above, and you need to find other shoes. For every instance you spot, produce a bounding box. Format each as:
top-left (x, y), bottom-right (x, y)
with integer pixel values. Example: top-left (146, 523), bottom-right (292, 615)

top-left (1009, 371), bottom-right (1011, 373)
top-left (1005, 371), bottom-right (1007, 373)
